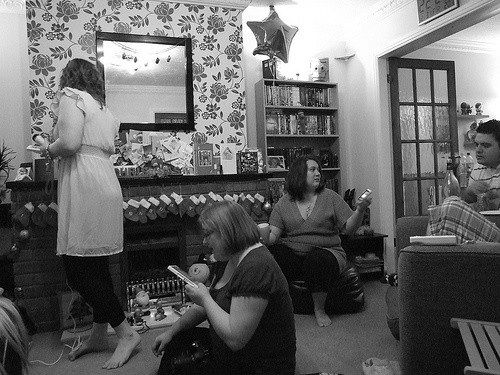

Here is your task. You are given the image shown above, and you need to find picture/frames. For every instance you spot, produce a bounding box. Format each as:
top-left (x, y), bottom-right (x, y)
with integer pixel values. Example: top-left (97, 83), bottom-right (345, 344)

top-left (266, 156), bottom-right (285, 170)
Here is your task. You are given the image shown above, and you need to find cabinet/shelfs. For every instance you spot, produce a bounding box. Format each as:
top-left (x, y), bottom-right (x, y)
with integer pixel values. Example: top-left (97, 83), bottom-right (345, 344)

top-left (341, 234), bottom-right (388, 280)
top-left (457, 114), bottom-right (489, 146)
top-left (253, 79), bottom-right (341, 205)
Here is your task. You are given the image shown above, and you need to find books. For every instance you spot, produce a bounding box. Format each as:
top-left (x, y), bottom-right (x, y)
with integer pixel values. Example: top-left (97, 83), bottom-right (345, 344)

top-left (167, 265), bottom-right (199, 289)
top-left (265, 83), bottom-right (337, 208)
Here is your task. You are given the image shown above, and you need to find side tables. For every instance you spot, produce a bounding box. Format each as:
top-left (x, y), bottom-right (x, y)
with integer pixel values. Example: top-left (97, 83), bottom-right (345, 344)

top-left (449, 319), bottom-right (500, 375)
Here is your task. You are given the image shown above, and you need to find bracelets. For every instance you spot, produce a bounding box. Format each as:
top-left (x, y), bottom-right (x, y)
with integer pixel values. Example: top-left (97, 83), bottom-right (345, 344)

top-left (45, 143), bottom-right (52, 160)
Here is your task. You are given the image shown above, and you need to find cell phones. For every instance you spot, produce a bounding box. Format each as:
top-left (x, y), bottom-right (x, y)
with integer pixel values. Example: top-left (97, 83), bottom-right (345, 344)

top-left (167, 265), bottom-right (199, 289)
top-left (357, 189), bottom-right (372, 204)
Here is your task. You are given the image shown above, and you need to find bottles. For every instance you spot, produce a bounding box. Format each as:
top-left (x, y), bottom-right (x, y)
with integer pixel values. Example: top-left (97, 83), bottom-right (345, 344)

top-left (440, 152), bottom-right (475, 200)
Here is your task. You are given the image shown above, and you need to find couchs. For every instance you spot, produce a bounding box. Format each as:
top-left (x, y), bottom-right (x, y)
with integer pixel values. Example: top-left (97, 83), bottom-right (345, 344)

top-left (395, 215), bottom-right (500, 375)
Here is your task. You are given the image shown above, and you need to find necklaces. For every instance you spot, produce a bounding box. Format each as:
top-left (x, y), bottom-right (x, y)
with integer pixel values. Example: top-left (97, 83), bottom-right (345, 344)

top-left (302, 193), bottom-right (317, 219)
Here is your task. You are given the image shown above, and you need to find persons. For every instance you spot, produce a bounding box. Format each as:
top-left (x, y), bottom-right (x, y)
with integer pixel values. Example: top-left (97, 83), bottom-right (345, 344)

top-left (385, 119), bottom-right (500, 287)
top-left (152, 202), bottom-right (298, 375)
top-left (251, 157), bottom-right (373, 327)
top-left (39, 59), bottom-right (141, 369)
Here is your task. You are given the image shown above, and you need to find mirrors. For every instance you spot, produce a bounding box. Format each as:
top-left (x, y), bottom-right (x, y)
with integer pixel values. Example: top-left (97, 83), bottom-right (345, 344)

top-left (96, 30), bottom-right (195, 131)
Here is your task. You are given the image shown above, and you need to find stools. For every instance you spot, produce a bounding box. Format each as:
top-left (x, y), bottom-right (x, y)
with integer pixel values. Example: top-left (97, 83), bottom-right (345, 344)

top-left (290, 264), bottom-right (365, 316)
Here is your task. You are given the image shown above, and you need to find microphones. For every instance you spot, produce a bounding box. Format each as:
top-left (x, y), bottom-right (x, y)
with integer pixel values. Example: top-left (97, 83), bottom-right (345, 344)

top-left (32, 134), bottom-right (45, 145)
